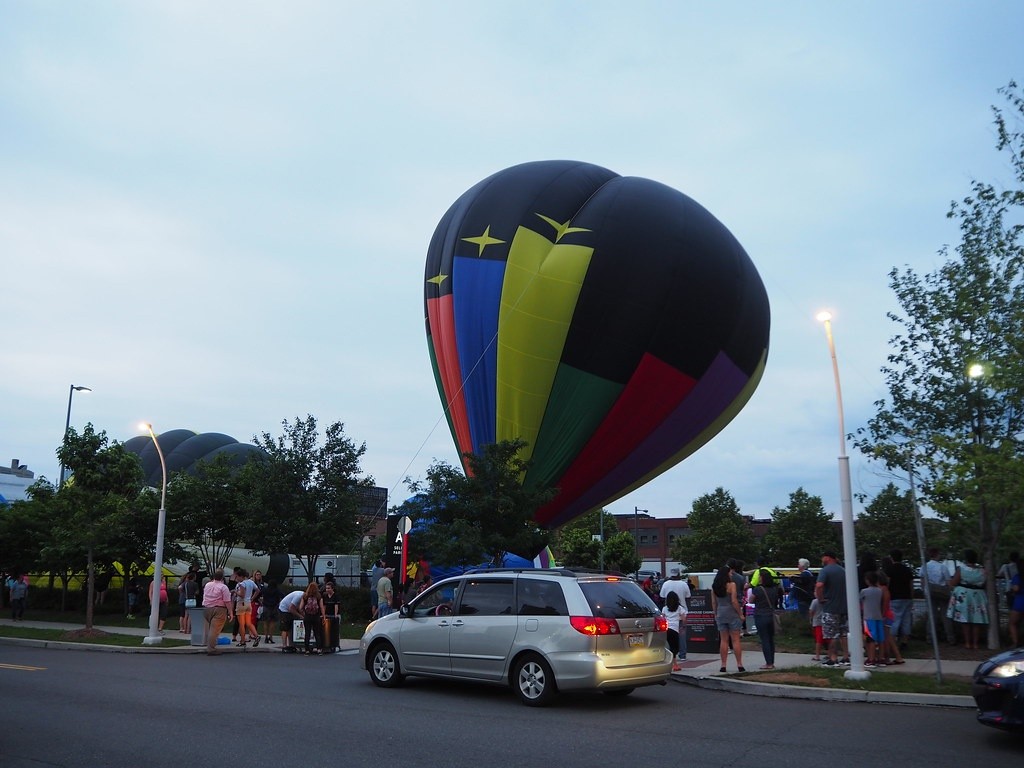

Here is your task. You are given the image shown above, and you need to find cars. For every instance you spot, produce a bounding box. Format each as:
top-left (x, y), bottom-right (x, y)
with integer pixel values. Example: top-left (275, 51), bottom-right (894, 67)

top-left (969, 647), bottom-right (1024, 731)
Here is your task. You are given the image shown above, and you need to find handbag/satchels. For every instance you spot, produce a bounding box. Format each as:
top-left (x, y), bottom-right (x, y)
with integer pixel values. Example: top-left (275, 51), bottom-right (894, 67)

top-left (773, 613), bottom-right (783, 634)
top-left (185, 599), bottom-right (196, 607)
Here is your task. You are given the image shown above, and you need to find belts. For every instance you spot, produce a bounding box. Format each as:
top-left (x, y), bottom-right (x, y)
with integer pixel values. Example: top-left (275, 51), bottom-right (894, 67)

top-left (958, 584), bottom-right (980, 589)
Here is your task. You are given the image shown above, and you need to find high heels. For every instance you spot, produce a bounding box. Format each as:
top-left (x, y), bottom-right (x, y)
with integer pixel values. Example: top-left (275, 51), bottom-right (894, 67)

top-left (265, 637), bottom-right (268, 643)
top-left (268, 637), bottom-right (276, 644)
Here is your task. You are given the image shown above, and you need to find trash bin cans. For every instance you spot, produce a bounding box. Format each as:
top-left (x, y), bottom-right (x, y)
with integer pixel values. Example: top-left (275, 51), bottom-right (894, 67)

top-left (188, 607), bottom-right (208, 646)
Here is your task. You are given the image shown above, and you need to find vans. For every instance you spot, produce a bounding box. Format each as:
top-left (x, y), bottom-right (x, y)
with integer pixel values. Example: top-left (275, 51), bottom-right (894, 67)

top-left (358, 567), bottom-right (675, 708)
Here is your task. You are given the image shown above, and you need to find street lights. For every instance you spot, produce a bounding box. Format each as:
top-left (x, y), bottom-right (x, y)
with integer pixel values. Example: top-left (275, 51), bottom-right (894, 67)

top-left (815, 310), bottom-right (871, 680)
top-left (48, 385), bottom-right (93, 593)
top-left (137, 421), bottom-right (166, 645)
top-left (635, 506), bottom-right (648, 580)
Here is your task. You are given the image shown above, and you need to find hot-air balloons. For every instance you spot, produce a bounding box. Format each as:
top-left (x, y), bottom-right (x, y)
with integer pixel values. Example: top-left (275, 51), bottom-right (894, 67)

top-left (18, 429), bottom-right (311, 591)
top-left (400, 160), bottom-right (770, 599)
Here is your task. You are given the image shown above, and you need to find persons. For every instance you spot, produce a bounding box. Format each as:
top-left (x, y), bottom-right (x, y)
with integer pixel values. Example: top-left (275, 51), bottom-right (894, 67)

top-left (231, 569), bottom-right (280, 648)
top-left (300, 582), bottom-right (326, 656)
top-left (947, 549), bottom-right (990, 648)
top-left (377, 568), bottom-right (395, 617)
top-left (279, 591), bottom-right (306, 647)
top-left (217, 567), bottom-right (251, 642)
top-left (998, 548), bottom-right (1024, 644)
top-left (368, 560), bottom-right (385, 623)
top-left (642, 567), bottom-right (696, 671)
top-left (177, 560), bottom-right (211, 634)
top-left (10, 577), bottom-right (28, 621)
top-left (320, 573), bottom-right (341, 623)
top-left (711, 559), bottom-right (786, 672)
top-left (127, 578), bottom-right (138, 619)
top-left (920, 549), bottom-right (956, 645)
top-left (789, 549), bottom-right (913, 668)
top-left (401, 575), bottom-right (433, 608)
top-left (149, 571), bottom-right (168, 634)
top-left (94, 576), bottom-right (106, 605)
top-left (202, 571), bottom-right (233, 655)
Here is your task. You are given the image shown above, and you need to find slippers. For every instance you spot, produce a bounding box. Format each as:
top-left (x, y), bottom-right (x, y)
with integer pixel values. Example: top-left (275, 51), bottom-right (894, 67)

top-left (892, 660), bottom-right (905, 664)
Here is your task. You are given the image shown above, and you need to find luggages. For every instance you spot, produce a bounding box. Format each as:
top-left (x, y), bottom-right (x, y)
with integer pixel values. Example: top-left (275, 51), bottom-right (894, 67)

top-left (322, 617), bottom-right (339, 649)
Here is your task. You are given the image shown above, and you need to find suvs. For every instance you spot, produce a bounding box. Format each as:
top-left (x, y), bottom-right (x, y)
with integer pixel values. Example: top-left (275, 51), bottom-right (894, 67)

top-left (626, 570), bottom-right (662, 584)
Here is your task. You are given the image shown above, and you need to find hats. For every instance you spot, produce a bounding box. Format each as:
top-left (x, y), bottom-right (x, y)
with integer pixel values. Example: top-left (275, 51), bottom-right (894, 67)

top-left (820, 552), bottom-right (836, 559)
top-left (383, 568), bottom-right (395, 573)
top-left (670, 567), bottom-right (679, 576)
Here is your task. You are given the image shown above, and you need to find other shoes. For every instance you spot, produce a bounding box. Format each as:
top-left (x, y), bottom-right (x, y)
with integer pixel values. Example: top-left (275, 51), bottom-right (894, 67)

top-left (206, 648), bottom-right (222, 655)
top-left (680, 657), bottom-right (685, 661)
top-left (673, 665), bottom-right (682, 671)
top-left (728, 647), bottom-right (731, 654)
top-left (743, 633), bottom-right (755, 636)
top-left (811, 658), bottom-right (819, 661)
top-left (232, 636), bottom-right (261, 647)
top-left (760, 665), bottom-right (773, 669)
top-left (159, 631), bottom-right (190, 635)
top-left (282, 647), bottom-right (340, 656)
top-left (720, 667), bottom-right (726, 671)
top-left (738, 667), bottom-right (745, 671)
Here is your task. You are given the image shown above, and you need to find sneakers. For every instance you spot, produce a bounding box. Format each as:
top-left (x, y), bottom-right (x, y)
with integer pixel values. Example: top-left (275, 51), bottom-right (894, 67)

top-left (839, 659), bottom-right (850, 665)
top-left (864, 662), bottom-right (876, 667)
top-left (878, 659), bottom-right (887, 667)
top-left (820, 660), bottom-right (839, 667)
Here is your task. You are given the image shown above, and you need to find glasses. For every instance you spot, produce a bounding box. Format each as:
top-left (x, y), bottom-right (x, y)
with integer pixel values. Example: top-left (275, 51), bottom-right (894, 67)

top-left (257, 574), bottom-right (260, 576)
